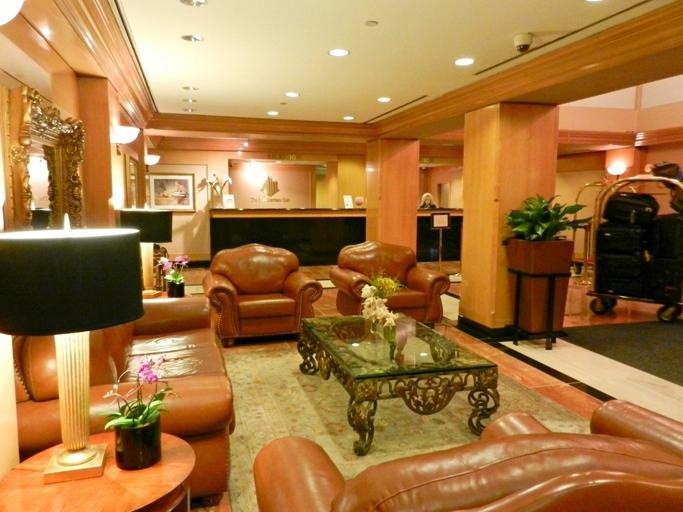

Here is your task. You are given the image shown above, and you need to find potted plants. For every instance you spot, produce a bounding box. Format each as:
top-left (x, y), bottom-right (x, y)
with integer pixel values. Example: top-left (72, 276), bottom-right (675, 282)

top-left (505, 194), bottom-right (595, 350)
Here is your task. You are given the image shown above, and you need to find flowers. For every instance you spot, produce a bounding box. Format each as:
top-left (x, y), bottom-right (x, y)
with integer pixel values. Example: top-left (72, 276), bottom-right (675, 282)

top-left (206, 172), bottom-right (231, 208)
top-left (162, 253), bottom-right (190, 284)
top-left (359, 270), bottom-right (413, 364)
top-left (104, 357), bottom-right (181, 436)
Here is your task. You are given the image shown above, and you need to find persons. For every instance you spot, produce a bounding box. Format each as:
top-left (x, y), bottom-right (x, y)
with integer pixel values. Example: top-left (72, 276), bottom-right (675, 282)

top-left (417, 193), bottom-right (436, 208)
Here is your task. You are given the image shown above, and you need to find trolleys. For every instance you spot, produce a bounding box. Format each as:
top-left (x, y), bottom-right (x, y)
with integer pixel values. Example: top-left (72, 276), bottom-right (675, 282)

top-left (587, 177), bottom-right (683, 323)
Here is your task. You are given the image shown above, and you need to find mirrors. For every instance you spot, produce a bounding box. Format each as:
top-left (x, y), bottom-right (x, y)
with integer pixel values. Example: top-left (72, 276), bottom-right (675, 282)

top-left (1, 83), bottom-right (85, 231)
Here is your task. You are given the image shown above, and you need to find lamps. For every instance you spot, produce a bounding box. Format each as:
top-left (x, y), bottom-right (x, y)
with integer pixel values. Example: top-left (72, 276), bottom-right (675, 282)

top-left (1, 228), bottom-right (145, 486)
top-left (107, 125), bottom-right (141, 155)
top-left (119, 207), bottom-right (172, 297)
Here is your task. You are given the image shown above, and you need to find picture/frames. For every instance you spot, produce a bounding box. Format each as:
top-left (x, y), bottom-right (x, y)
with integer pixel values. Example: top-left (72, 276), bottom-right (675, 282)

top-left (149, 172), bottom-right (194, 212)
top-left (125, 154), bottom-right (139, 206)
top-left (145, 154), bottom-right (160, 172)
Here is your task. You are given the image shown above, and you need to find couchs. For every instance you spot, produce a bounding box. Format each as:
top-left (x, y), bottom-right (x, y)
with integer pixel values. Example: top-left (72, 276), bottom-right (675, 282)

top-left (253, 399), bottom-right (683, 512)
top-left (13, 290), bottom-right (236, 505)
top-left (202, 244), bottom-right (324, 347)
top-left (329, 240), bottom-right (451, 329)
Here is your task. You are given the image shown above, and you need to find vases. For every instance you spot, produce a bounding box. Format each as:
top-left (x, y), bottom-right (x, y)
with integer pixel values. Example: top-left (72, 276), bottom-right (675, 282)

top-left (168, 283), bottom-right (186, 295)
top-left (114, 414), bottom-right (161, 471)
